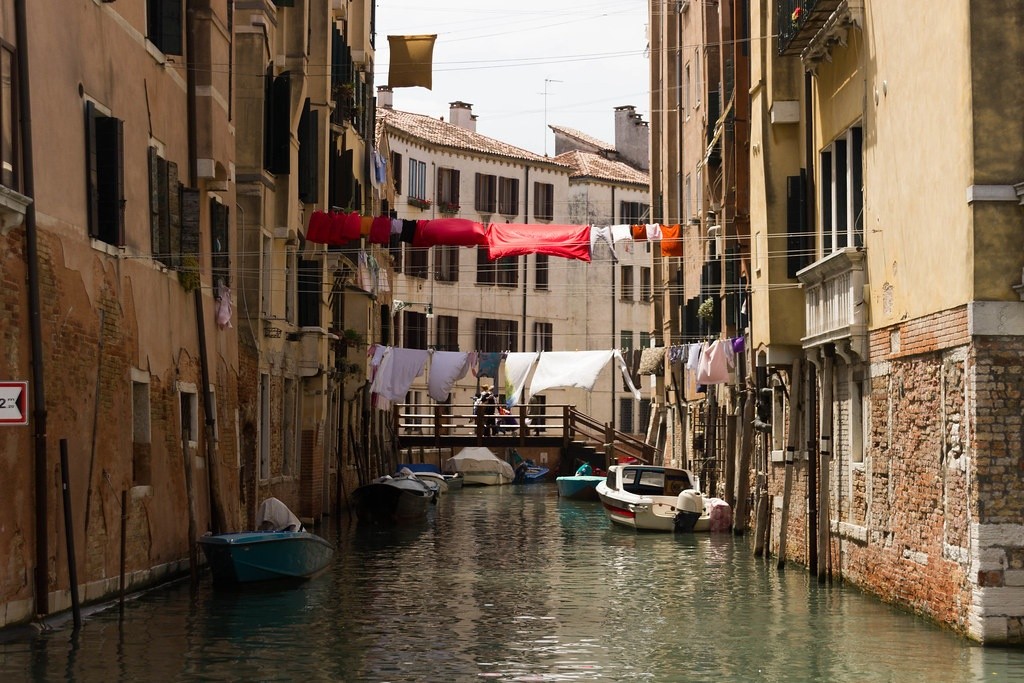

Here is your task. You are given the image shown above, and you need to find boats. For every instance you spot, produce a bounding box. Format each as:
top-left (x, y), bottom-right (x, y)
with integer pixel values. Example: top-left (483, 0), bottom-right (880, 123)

top-left (351, 475), bottom-right (440, 522)
top-left (443, 446), bottom-right (516, 486)
top-left (595, 464), bottom-right (714, 534)
top-left (556, 474), bottom-right (608, 500)
top-left (413, 472), bottom-right (448, 494)
top-left (194, 531), bottom-right (332, 585)
top-left (520, 465), bottom-right (551, 483)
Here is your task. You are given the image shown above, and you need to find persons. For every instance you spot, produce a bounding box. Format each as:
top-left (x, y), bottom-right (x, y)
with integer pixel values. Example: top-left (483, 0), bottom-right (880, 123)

top-left (528, 392), bottom-right (540, 436)
top-left (469, 385), bottom-right (501, 435)
